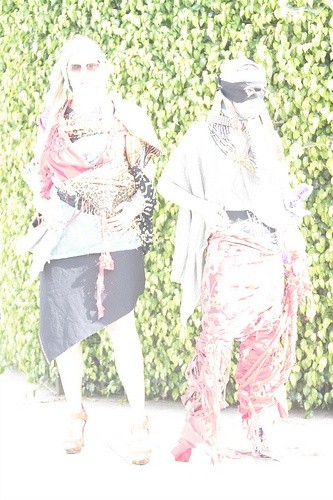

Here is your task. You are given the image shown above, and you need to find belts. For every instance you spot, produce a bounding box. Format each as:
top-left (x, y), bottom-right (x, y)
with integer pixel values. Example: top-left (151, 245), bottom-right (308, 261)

top-left (225, 210), bottom-right (258, 222)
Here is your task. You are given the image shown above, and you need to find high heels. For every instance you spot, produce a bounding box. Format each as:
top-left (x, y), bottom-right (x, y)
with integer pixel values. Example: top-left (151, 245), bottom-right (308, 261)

top-left (131, 416), bottom-right (151, 465)
top-left (64, 403), bottom-right (88, 454)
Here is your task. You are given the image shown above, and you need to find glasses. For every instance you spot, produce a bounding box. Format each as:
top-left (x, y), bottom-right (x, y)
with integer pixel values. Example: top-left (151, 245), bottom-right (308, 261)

top-left (68, 63), bottom-right (103, 74)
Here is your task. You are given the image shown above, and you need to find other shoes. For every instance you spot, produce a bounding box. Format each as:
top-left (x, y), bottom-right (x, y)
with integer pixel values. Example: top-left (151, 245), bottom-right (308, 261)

top-left (253, 444), bottom-right (303, 466)
top-left (186, 448), bottom-right (214, 487)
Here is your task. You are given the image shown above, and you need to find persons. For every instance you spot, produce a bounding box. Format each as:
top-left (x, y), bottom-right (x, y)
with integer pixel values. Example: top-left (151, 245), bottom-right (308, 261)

top-left (157, 56), bottom-right (315, 465)
top-left (12, 32), bottom-right (163, 466)
